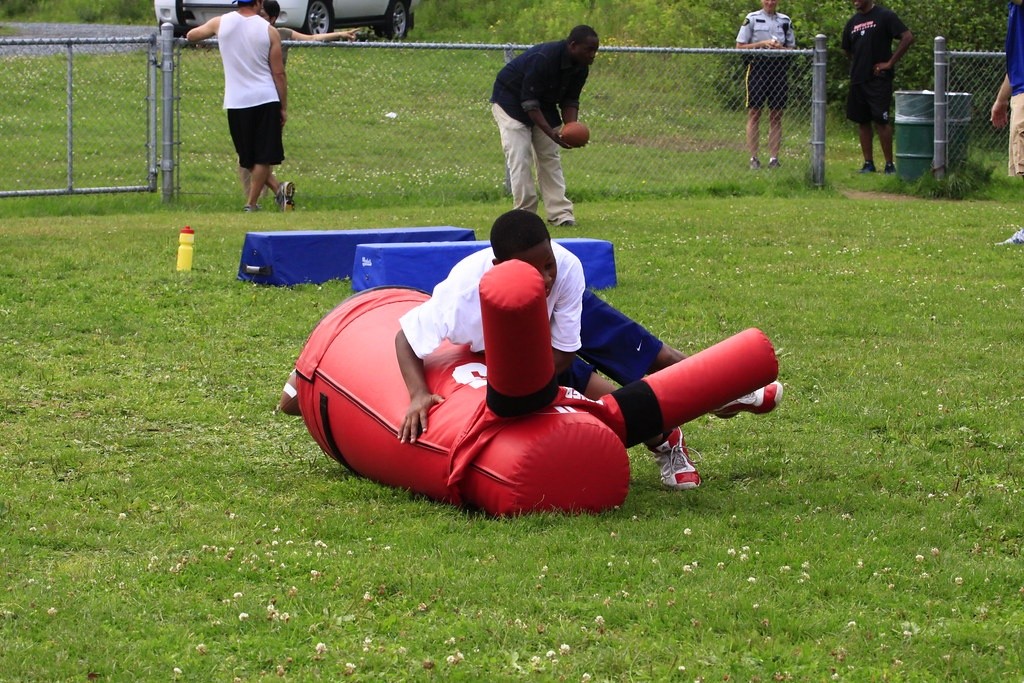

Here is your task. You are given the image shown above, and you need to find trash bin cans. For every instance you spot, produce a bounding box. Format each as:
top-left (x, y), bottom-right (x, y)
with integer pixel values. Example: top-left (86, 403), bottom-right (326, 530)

top-left (893, 91), bottom-right (973, 182)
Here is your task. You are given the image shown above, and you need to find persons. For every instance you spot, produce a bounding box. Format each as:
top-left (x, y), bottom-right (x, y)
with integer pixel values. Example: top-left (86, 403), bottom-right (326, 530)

top-left (990, 0), bottom-right (1024, 247)
top-left (394, 206), bottom-right (783, 490)
top-left (840, 0), bottom-right (913, 174)
top-left (489, 24), bottom-right (599, 225)
top-left (736, 0), bottom-right (795, 171)
top-left (239, 0), bottom-right (362, 202)
top-left (187, 0), bottom-right (294, 215)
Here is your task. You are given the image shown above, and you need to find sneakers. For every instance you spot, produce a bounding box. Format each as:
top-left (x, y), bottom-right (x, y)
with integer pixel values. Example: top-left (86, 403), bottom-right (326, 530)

top-left (274, 181), bottom-right (294, 211)
top-left (647, 425), bottom-right (701, 490)
top-left (244, 203), bottom-right (260, 212)
top-left (711, 382), bottom-right (783, 419)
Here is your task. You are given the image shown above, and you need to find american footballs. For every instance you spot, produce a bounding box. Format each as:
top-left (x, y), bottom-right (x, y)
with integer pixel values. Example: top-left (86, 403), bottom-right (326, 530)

top-left (561, 122), bottom-right (589, 146)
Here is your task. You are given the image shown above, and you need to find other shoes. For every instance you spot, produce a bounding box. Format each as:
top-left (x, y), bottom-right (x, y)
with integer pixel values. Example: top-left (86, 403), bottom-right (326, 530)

top-left (885, 163), bottom-right (896, 174)
top-left (859, 164), bottom-right (876, 175)
top-left (768, 159), bottom-right (780, 168)
top-left (994, 232), bottom-right (1024, 245)
top-left (561, 220), bottom-right (574, 228)
top-left (750, 158), bottom-right (761, 169)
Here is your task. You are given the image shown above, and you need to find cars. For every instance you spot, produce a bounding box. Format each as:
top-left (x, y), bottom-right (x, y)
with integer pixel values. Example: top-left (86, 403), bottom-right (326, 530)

top-left (153, 0), bottom-right (419, 49)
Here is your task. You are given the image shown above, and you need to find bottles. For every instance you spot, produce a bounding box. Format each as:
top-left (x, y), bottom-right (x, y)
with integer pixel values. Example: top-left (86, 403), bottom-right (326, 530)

top-left (176, 225), bottom-right (195, 271)
